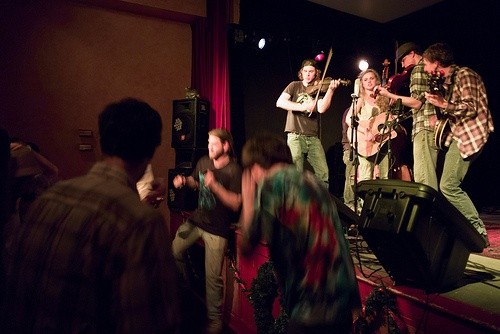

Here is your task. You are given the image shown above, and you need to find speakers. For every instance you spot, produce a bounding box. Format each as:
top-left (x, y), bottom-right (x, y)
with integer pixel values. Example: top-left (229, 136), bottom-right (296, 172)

top-left (354, 180), bottom-right (486, 291)
top-left (171, 98), bottom-right (210, 149)
top-left (167, 169), bottom-right (200, 212)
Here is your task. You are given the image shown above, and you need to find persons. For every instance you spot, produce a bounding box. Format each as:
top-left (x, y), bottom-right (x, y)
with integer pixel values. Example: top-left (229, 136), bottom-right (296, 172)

top-left (423, 42), bottom-right (494, 248)
top-left (342, 42), bottom-right (444, 229)
top-left (0, 97), bottom-right (179, 334)
top-left (276, 60), bottom-right (341, 192)
top-left (0, 127), bottom-right (168, 301)
top-left (238, 134), bottom-right (361, 334)
top-left (173, 128), bottom-right (244, 334)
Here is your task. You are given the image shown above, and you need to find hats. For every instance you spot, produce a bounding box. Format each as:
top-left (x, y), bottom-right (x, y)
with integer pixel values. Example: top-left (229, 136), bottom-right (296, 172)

top-left (211, 127), bottom-right (234, 157)
top-left (396, 42), bottom-right (416, 61)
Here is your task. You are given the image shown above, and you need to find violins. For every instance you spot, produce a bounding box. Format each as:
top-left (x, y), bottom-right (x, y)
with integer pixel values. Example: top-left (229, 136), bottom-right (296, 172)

top-left (371, 63), bottom-right (416, 98)
top-left (306, 77), bottom-right (350, 96)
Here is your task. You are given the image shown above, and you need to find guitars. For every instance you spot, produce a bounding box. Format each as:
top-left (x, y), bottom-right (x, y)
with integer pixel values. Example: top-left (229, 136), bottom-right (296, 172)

top-left (426, 70), bottom-right (451, 150)
top-left (347, 110), bottom-right (412, 158)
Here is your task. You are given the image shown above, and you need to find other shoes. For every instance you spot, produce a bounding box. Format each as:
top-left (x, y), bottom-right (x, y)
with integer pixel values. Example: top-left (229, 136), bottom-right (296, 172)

top-left (481, 234), bottom-right (490, 245)
top-left (209, 319), bottom-right (222, 333)
top-left (348, 228), bottom-right (357, 236)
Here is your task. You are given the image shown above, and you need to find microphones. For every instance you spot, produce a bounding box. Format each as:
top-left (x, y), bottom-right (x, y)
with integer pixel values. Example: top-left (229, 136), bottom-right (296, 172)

top-left (394, 98), bottom-right (403, 124)
top-left (354, 79), bottom-right (361, 104)
